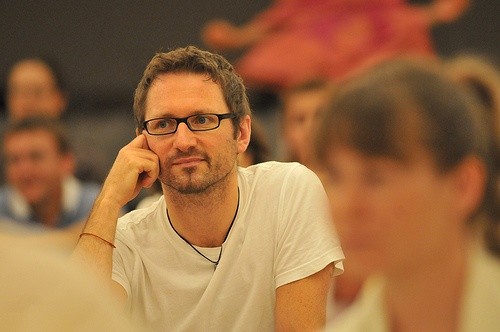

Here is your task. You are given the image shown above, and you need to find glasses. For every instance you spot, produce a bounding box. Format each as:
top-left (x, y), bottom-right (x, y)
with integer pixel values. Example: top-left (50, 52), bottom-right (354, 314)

top-left (142, 111), bottom-right (236, 136)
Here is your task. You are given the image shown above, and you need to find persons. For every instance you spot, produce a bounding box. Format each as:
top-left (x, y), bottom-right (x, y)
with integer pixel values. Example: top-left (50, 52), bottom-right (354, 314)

top-left (0, 115), bottom-right (94, 230)
top-left (70, 45), bottom-right (346, 332)
top-left (200, 0), bottom-right (468, 163)
top-left (234, 116), bottom-right (274, 171)
top-left (273, 75), bottom-right (375, 317)
top-left (303, 50), bottom-right (500, 332)
top-left (0, 53), bottom-right (126, 235)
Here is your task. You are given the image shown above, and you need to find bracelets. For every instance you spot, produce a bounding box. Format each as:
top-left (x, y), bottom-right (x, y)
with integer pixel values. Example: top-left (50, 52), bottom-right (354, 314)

top-left (79, 233), bottom-right (117, 249)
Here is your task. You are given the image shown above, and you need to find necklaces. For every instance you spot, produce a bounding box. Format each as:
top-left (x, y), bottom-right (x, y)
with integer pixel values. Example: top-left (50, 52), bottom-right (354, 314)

top-left (166, 187), bottom-right (240, 273)
